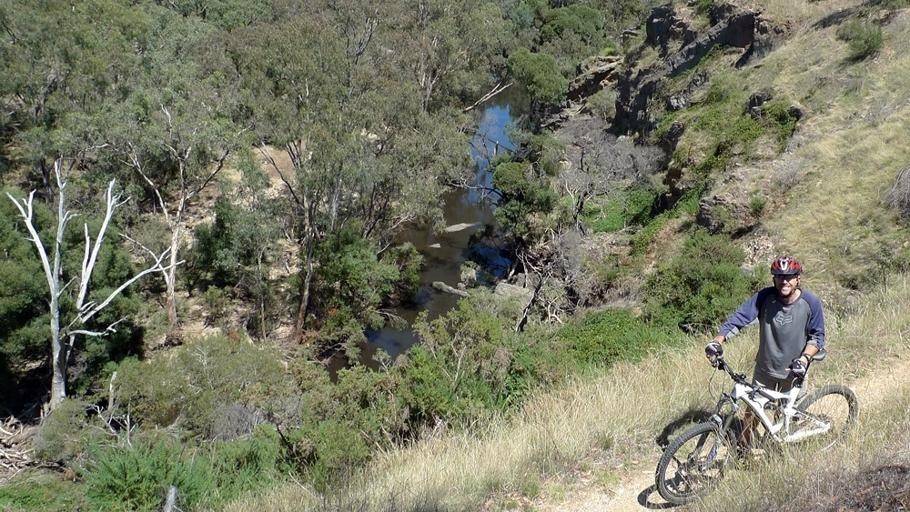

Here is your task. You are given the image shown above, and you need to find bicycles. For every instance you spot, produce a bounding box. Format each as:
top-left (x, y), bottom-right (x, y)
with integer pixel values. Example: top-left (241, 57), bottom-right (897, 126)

top-left (654, 345), bottom-right (859, 506)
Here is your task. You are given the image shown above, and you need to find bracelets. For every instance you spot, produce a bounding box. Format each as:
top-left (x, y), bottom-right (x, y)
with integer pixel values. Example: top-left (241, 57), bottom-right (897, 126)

top-left (803, 354), bottom-right (812, 362)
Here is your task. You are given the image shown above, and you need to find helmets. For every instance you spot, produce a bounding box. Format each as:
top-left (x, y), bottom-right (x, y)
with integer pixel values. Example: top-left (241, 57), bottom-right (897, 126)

top-left (771, 255), bottom-right (801, 275)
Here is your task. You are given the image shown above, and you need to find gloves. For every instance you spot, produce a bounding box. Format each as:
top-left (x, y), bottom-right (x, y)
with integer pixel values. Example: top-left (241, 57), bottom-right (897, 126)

top-left (704, 340), bottom-right (723, 363)
top-left (789, 353), bottom-right (812, 377)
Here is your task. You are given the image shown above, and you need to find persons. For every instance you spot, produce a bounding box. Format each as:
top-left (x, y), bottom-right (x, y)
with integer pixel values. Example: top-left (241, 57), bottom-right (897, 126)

top-left (710, 255), bottom-right (826, 472)
